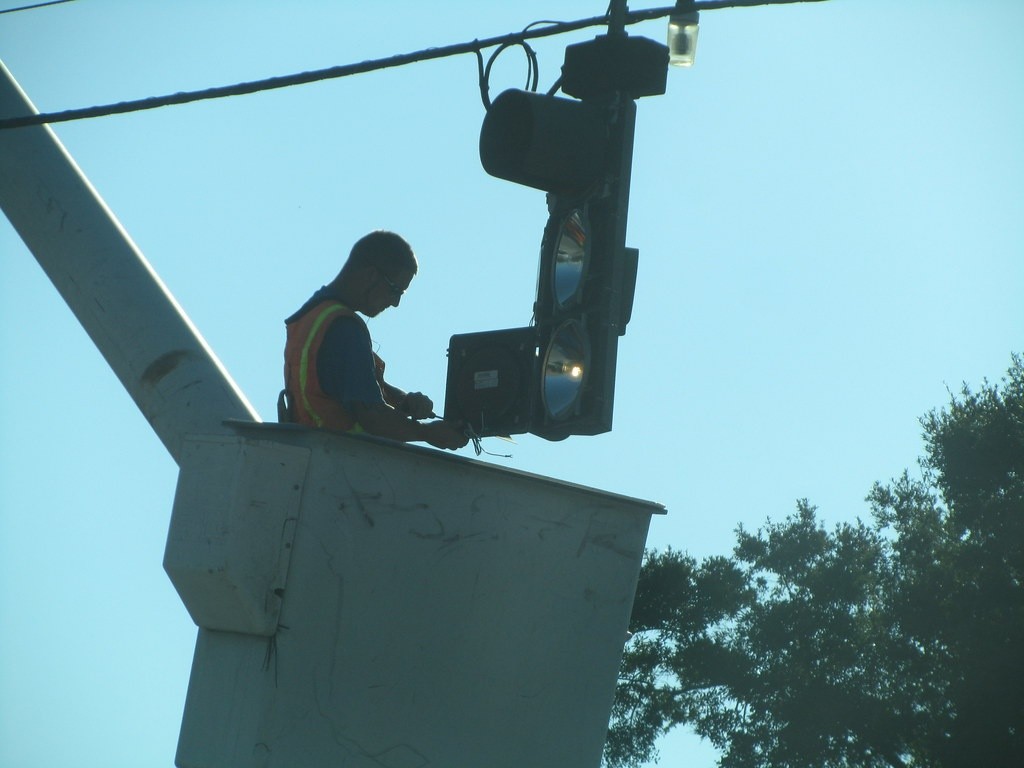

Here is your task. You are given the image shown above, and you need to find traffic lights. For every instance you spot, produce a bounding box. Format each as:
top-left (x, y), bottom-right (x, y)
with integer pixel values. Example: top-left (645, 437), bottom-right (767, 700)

top-left (478, 89), bottom-right (640, 442)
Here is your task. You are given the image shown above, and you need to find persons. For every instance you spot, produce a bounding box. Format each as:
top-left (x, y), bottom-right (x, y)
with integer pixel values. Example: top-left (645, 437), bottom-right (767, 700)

top-left (285, 231), bottom-right (468, 450)
